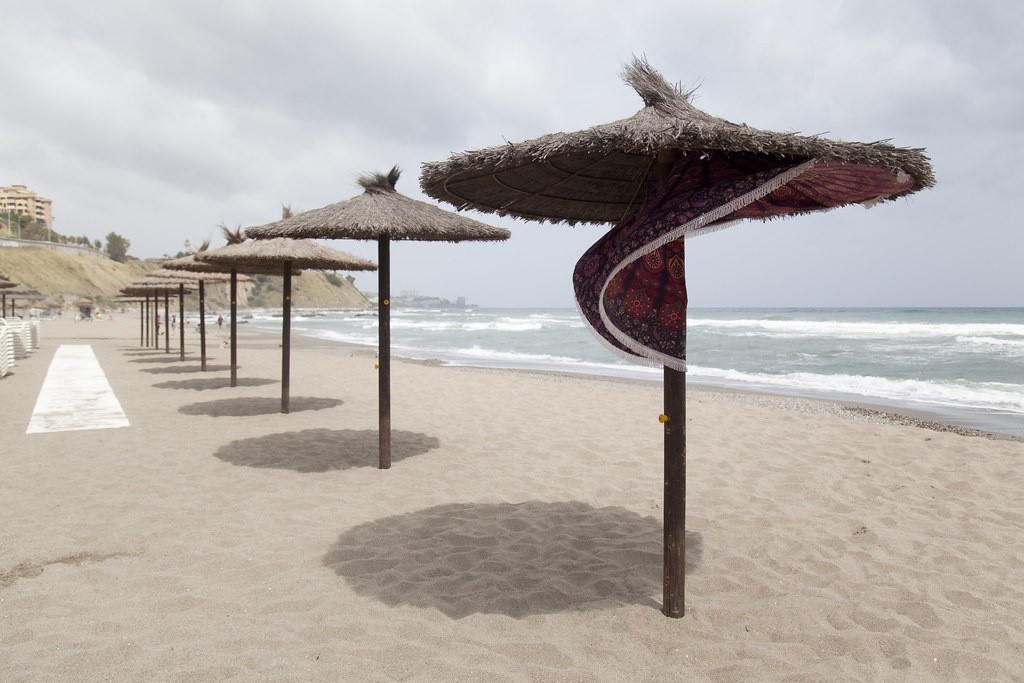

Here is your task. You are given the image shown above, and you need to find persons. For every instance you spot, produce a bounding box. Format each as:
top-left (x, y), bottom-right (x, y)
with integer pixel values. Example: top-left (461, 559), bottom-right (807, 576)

top-left (158, 315), bottom-right (163, 334)
top-left (218, 315), bottom-right (223, 327)
top-left (96, 313), bottom-right (102, 319)
top-left (172, 315), bottom-right (175, 330)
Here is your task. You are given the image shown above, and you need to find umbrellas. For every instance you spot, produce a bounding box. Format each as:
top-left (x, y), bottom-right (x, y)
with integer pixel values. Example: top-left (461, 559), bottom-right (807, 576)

top-left (419, 59), bottom-right (936, 618)
top-left (147, 268), bottom-right (254, 371)
top-left (0, 269), bottom-right (43, 318)
top-left (114, 284), bottom-right (198, 353)
top-left (195, 207), bottom-right (379, 414)
top-left (75, 298), bottom-right (92, 312)
top-left (162, 226), bottom-right (300, 390)
top-left (246, 169), bottom-right (511, 468)
top-left (132, 277), bottom-right (224, 362)
